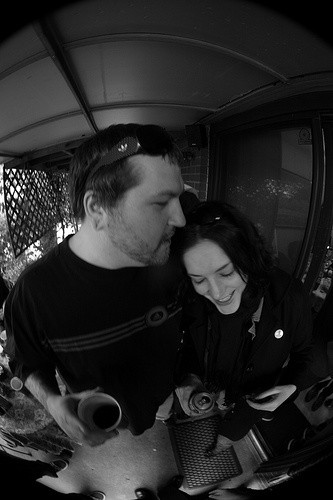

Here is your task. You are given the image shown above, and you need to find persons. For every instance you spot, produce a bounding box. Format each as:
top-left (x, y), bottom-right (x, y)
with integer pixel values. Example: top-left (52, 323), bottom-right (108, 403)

top-left (4, 124), bottom-right (240, 447)
top-left (166, 198), bottom-right (332, 423)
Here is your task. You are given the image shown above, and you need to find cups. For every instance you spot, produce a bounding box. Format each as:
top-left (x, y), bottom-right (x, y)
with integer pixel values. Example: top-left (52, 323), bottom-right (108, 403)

top-left (77, 392), bottom-right (131, 434)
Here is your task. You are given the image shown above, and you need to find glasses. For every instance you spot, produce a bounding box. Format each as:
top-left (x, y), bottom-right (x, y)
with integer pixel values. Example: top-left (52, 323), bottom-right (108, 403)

top-left (173, 200), bottom-right (256, 235)
top-left (87, 122), bottom-right (186, 181)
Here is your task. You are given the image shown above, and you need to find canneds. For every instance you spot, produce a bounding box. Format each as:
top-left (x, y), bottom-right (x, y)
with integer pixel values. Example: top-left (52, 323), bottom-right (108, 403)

top-left (188, 392), bottom-right (215, 414)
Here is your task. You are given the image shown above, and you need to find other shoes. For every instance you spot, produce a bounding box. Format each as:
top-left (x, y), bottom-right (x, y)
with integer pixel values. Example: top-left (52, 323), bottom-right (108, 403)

top-left (301, 424), bottom-right (323, 444)
top-left (88, 491), bottom-right (106, 500)
top-left (134, 488), bottom-right (157, 500)
top-left (47, 459), bottom-right (68, 474)
top-left (288, 437), bottom-right (304, 451)
top-left (159, 485), bottom-right (179, 499)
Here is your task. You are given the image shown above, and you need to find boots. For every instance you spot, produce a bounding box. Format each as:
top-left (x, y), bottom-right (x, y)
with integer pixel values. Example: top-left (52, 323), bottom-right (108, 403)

top-left (305, 379), bottom-right (333, 403)
top-left (202, 433), bottom-right (245, 459)
top-left (311, 381), bottom-right (333, 412)
